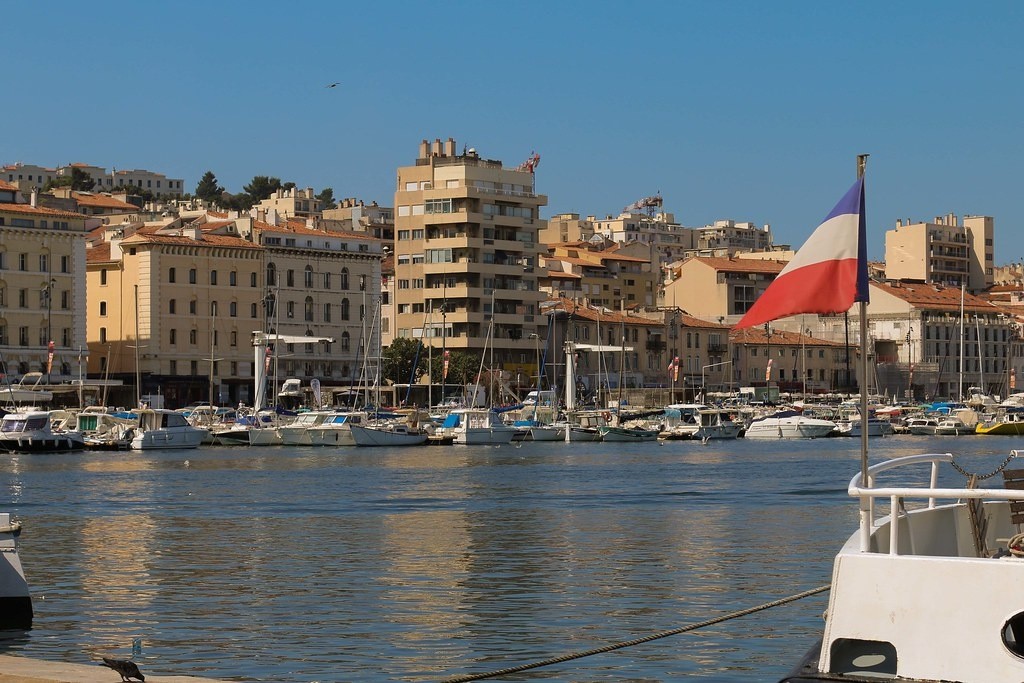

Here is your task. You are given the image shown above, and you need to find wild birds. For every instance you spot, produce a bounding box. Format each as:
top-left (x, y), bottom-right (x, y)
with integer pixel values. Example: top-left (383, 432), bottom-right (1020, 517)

top-left (323, 82), bottom-right (341, 88)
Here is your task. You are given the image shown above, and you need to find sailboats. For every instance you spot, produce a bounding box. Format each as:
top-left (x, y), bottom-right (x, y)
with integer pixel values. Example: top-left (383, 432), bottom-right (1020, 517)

top-left (0, 262), bottom-right (1024, 454)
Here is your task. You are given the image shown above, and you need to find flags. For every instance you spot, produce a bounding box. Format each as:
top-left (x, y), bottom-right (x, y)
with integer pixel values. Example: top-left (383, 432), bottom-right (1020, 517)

top-left (734, 173), bottom-right (871, 331)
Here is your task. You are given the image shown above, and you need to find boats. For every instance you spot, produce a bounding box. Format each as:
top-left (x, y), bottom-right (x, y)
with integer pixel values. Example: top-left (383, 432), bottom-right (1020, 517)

top-left (0, 513), bottom-right (35, 632)
top-left (773, 154), bottom-right (1024, 683)
